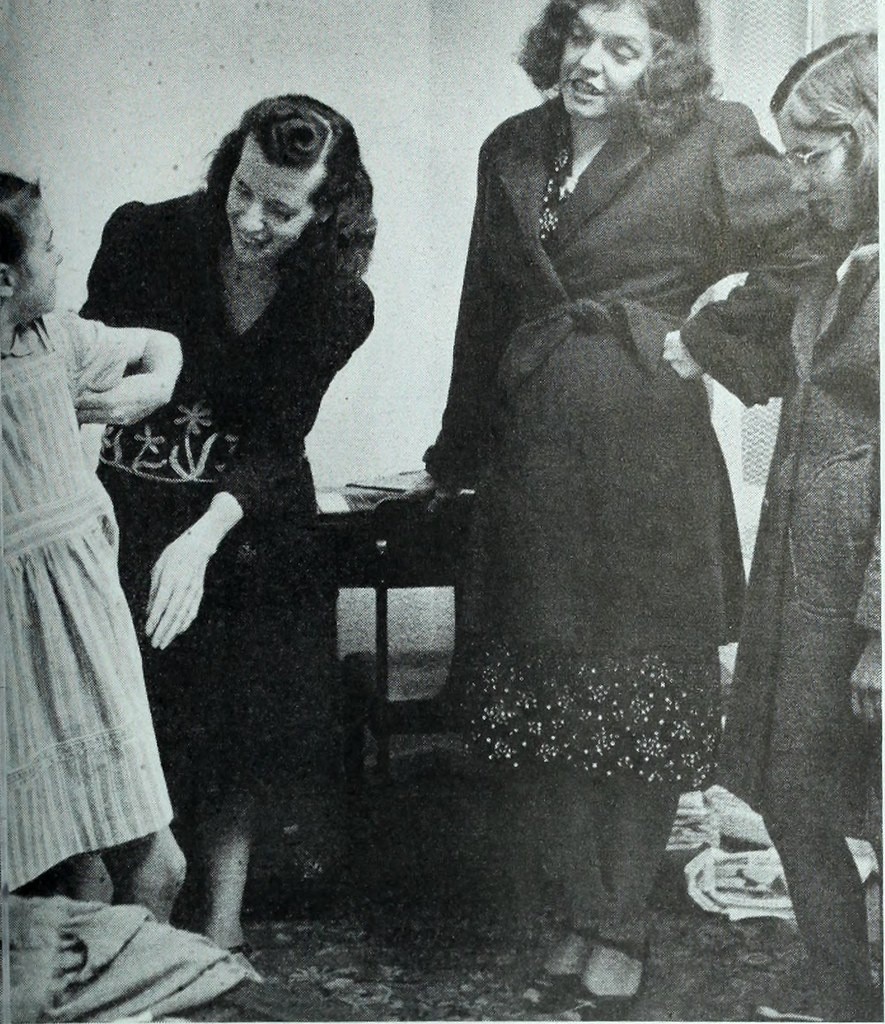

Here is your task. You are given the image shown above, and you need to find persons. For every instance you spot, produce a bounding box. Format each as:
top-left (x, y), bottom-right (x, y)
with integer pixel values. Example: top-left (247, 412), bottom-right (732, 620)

top-left (405, 0), bottom-right (814, 1022)
top-left (0, 174), bottom-right (185, 926)
top-left (81, 92), bottom-right (375, 982)
top-left (723, 34), bottom-right (884, 1022)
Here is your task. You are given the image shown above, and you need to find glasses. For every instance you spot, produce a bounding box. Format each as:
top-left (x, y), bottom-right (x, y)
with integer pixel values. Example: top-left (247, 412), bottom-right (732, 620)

top-left (779, 104), bottom-right (870, 181)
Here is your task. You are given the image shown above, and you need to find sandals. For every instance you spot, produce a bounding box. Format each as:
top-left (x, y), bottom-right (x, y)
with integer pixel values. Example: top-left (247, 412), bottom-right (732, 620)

top-left (557, 931), bottom-right (648, 1022)
top-left (522, 924), bottom-right (591, 1011)
